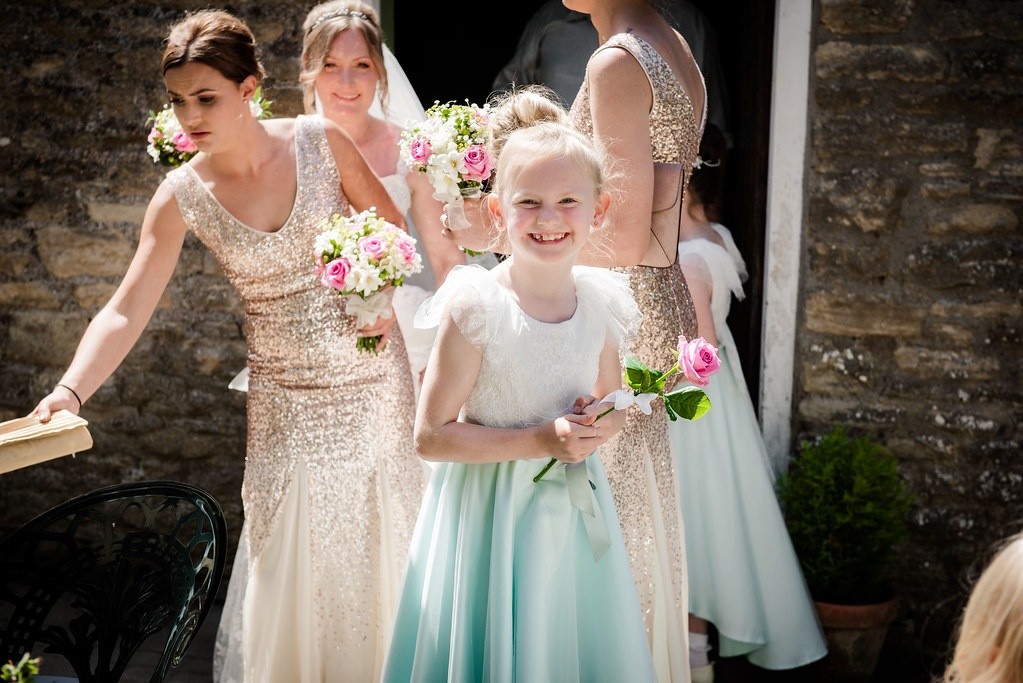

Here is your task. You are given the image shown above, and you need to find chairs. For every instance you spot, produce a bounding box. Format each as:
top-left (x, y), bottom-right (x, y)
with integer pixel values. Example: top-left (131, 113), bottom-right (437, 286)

top-left (0, 480), bottom-right (229, 683)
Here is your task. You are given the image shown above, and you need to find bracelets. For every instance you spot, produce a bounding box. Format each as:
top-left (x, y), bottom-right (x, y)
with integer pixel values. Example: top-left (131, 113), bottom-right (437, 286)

top-left (54, 385), bottom-right (81, 409)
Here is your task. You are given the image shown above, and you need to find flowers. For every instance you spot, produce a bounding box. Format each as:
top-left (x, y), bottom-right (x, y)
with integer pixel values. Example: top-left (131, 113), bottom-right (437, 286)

top-left (144, 87), bottom-right (273, 168)
top-left (533, 335), bottom-right (721, 484)
top-left (312, 207), bottom-right (424, 359)
top-left (396, 99), bottom-right (497, 258)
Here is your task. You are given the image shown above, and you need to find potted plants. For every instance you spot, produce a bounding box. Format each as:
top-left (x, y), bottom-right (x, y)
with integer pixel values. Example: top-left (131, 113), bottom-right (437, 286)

top-left (768, 426), bottom-right (914, 676)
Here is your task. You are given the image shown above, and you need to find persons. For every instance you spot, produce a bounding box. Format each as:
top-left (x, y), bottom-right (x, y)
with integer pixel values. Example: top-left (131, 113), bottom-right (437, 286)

top-left (437, 0), bottom-right (708, 683)
top-left (675, 128), bottom-right (828, 683)
top-left (30, 12), bottom-right (429, 683)
top-left (381, 90), bottom-right (658, 683)
top-left (213, 0), bottom-right (503, 683)
top-left (944, 535), bottom-right (1023, 683)
top-left (491, 0), bottom-right (704, 113)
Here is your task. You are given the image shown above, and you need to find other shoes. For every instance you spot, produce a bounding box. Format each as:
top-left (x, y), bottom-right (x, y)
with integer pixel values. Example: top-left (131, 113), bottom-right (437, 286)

top-left (690, 661), bottom-right (717, 683)
